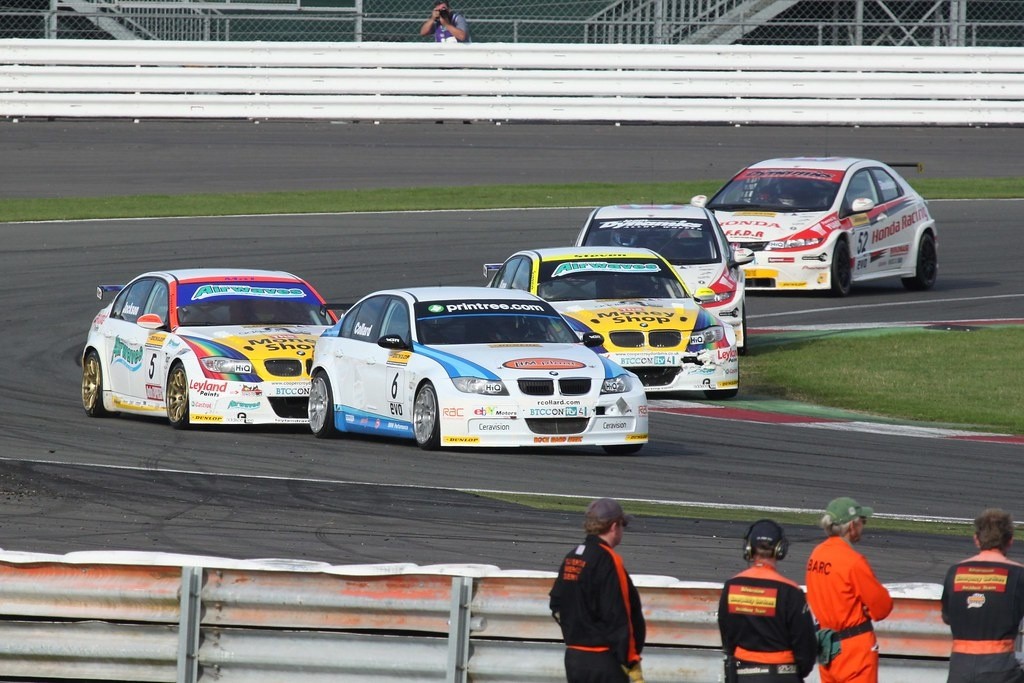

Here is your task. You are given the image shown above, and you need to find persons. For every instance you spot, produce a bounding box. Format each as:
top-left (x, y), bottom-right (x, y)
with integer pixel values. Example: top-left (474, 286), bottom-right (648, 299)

top-left (484, 314), bottom-right (529, 343)
top-left (717, 518), bottom-right (818, 683)
top-left (612, 273), bottom-right (649, 299)
top-left (548, 497), bottom-right (647, 683)
top-left (806, 496), bottom-right (895, 683)
top-left (420, 0), bottom-right (473, 125)
top-left (940, 506), bottom-right (1024, 683)
top-left (753, 184), bottom-right (784, 206)
top-left (610, 227), bottom-right (641, 248)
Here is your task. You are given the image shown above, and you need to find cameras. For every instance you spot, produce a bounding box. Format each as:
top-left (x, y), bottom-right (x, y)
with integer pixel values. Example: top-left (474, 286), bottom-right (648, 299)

top-left (435, 8), bottom-right (450, 19)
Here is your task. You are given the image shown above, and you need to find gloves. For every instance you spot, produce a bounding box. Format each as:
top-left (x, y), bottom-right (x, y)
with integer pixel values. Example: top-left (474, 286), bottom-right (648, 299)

top-left (622, 662), bottom-right (644, 683)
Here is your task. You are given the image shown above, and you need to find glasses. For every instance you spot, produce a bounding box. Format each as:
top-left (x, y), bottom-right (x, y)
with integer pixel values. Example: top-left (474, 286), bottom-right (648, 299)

top-left (615, 521), bottom-right (629, 527)
top-left (853, 516), bottom-right (868, 524)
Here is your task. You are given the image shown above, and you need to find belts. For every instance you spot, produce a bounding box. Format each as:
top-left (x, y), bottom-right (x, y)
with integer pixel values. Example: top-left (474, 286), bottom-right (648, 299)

top-left (830, 618), bottom-right (873, 645)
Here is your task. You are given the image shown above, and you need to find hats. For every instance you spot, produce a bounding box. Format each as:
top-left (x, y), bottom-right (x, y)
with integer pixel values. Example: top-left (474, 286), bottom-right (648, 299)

top-left (825, 497), bottom-right (875, 525)
top-left (750, 522), bottom-right (781, 544)
top-left (588, 498), bottom-right (632, 523)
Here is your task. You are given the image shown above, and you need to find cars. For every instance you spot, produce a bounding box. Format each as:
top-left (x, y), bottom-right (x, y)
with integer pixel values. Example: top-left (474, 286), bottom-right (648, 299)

top-left (81, 268), bottom-right (339, 431)
top-left (482, 246), bottom-right (739, 399)
top-left (573, 205), bottom-right (755, 356)
top-left (690, 156), bottom-right (938, 296)
top-left (307, 286), bottom-right (648, 454)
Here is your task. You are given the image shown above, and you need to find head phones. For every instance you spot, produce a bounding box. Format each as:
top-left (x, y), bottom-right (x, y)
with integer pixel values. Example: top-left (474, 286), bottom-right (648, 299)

top-left (742, 519), bottom-right (789, 561)
